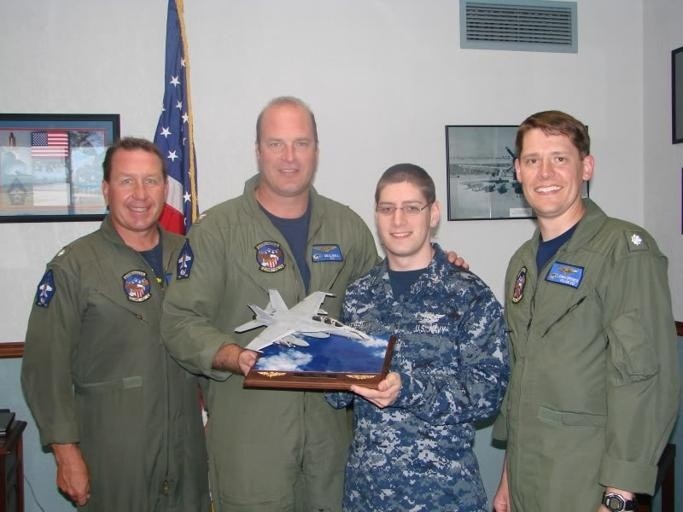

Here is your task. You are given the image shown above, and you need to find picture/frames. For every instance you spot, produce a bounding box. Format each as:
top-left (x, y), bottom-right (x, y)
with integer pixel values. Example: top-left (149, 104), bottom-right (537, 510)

top-left (445, 124), bottom-right (590, 222)
top-left (671, 46), bottom-right (683, 145)
top-left (0, 113), bottom-right (121, 223)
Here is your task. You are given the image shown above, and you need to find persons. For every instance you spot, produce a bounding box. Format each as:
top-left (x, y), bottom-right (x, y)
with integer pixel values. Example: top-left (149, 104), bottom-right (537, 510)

top-left (311, 157), bottom-right (518, 512)
top-left (156, 94), bottom-right (471, 512)
top-left (19, 135), bottom-right (213, 512)
top-left (476, 110), bottom-right (682, 511)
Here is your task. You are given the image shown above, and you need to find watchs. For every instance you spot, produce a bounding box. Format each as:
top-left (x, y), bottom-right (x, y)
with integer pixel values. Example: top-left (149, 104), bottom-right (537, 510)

top-left (600, 491), bottom-right (634, 510)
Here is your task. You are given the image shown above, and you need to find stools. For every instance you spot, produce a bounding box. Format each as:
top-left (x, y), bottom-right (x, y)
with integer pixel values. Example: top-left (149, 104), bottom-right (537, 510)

top-left (633, 443), bottom-right (676, 512)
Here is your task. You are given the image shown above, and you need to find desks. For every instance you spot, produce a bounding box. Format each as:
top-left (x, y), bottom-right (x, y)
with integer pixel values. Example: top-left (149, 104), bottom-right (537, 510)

top-left (0, 420), bottom-right (27, 512)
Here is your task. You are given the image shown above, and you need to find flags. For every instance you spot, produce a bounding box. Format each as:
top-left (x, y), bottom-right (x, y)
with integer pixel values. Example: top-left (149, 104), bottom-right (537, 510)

top-left (146, 2), bottom-right (211, 285)
top-left (28, 131), bottom-right (69, 160)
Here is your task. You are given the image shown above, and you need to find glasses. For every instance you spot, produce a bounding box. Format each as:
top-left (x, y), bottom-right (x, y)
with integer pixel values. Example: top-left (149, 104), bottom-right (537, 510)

top-left (375, 201), bottom-right (429, 216)
